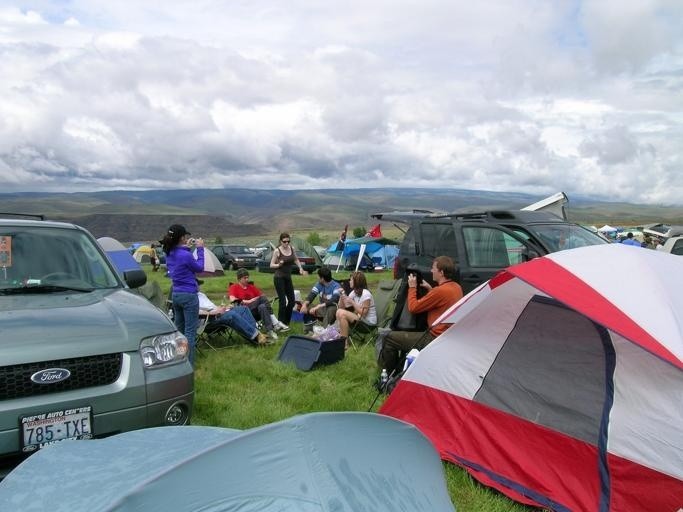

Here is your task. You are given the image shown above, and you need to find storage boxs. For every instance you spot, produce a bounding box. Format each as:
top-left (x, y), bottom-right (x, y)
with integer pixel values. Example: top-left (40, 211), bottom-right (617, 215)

top-left (278, 335), bottom-right (346, 372)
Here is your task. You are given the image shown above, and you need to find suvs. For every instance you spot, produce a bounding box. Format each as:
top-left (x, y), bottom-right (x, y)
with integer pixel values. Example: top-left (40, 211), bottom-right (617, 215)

top-left (371, 207), bottom-right (612, 297)
top-left (209, 245), bottom-right (258, 270)
top-left (0, 212), bottom-right (195, 457)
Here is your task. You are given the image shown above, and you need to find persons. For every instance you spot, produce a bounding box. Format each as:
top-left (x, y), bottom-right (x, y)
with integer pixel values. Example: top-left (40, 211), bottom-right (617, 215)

top-left (151, 243), bottom-right (160, 272)
top-left (161, 224), bottom-right (205, 369)
top-left (194, 278), bottom-right (275, 346)
top-left (375, 255), bottom-right (463, 395)
top-left (336, 271), bottom-right (378, 350)
top-left (611, 232), bottom-right (666, 250)
top-left (300, 267), bottom-right (342, 329)
top-left (229, 268), bottom-right (289, 339)
top-left (270, 233), bottom-right (304, 333)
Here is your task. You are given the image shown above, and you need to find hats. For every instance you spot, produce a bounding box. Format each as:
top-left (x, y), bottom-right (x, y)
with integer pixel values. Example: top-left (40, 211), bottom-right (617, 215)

top-left (168, 225), bottom-right (191, 238)
top-left (237, 269), bottom-right (248, 279)
top-left (304, 314), bottom-right (318, 326)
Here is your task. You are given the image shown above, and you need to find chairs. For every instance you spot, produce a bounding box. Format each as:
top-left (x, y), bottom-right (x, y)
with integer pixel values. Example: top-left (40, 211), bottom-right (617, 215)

top-left (344, 278), bottom-right (402, 352)
top-left (167, 279), bottom-right (278, 356)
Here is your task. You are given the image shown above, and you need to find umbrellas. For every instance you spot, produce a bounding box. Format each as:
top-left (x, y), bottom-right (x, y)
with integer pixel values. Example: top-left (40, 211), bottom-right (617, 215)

top-left (188, 245), bottom-right (225, 277)
top-left (597, 225), bottom-right (617, 235)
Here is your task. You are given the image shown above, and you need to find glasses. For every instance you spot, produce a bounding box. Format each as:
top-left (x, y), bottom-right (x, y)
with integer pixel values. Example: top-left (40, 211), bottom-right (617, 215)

top-left (282, 240), bottom-right (290, 243)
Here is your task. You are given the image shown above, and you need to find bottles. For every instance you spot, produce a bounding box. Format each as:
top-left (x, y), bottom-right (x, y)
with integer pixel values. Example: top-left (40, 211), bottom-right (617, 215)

top-left (341, 291), bottom-right (352, 309)
top-left (168, 309), bottom-right (173, 321)
top-left (380, 368), bottom-right (388, 383)
top-left (220, 294), bottom-right (227, 310)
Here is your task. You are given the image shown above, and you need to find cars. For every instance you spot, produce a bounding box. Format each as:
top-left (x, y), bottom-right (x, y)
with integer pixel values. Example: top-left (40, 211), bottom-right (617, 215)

top-left (254, 247), bottom-right (316, 274)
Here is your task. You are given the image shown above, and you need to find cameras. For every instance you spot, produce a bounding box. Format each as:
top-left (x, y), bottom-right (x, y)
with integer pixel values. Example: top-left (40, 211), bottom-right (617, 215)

top-left (191, 239), bottom-right (196, 245)
top-left (414, 271), bottom-right (422, 283)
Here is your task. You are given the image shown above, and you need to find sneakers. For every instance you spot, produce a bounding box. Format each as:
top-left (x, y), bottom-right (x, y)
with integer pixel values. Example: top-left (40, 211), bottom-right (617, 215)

top-left (255, 321), bottom-right (291, 344)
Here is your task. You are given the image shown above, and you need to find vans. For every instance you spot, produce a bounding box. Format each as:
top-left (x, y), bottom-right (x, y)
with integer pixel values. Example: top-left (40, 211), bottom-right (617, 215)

top-left (644, 223), bottom-right (683, 256)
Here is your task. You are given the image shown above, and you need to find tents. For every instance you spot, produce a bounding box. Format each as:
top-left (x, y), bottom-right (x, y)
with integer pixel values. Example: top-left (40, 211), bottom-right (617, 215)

top-left (378, 243), bottom-right (683, 511)
top-left (262, 236), bottom-right (400, 270)
top-left (128, 241), bottom-right (152, 264)
top-left (96, 237), bottom-right (142, 278)
top-left (0, 410), bottom-right (454, 512)
